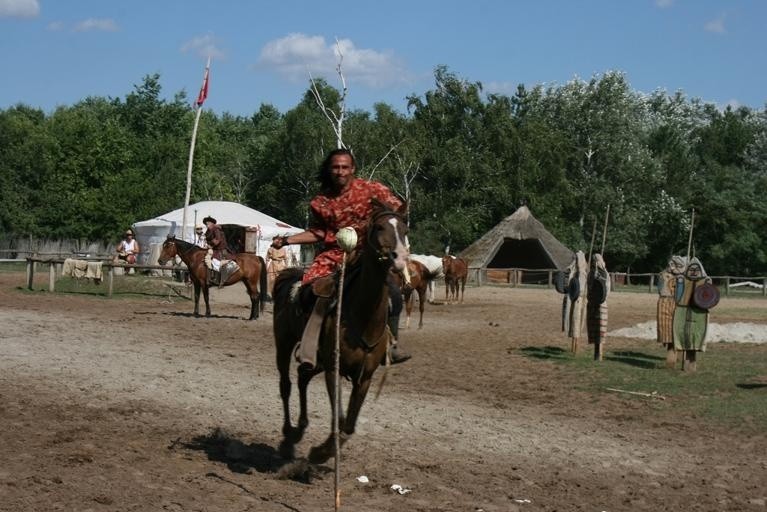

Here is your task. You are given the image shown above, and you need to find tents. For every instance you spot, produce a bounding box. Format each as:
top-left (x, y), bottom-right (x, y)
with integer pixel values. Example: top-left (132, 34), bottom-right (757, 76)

top-left (129, 199), bottom-right (308, 283)
top-left (451, 205), bottom-right (578, 279)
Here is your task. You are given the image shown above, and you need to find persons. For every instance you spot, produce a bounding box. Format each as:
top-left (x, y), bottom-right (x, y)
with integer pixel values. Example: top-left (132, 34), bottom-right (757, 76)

top-left (201, 215), bottom-right (229, 287)
top-left (265, 233), bottom-right (291, 301)
top-left (656, 253), bottom-right (687, 370)
top-left (270, 146), bottom-right (412, 374)
top-left (672, 256), bottom-right (720, 370)
top-left (585, 252), bottom-right (613, 362)
top-left (558, 248), bottom-right (588, 354)
top-left (115, 228), bottom-right (140, 276)
top-left (194, 225), bottom-right (207, 248)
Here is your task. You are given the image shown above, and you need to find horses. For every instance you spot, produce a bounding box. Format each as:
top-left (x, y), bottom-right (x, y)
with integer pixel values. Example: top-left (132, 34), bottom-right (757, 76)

top-left (441, 255), bottom-right (468, 305)
top-left (270, 195), bottom-right (413, 464)
top-left (157, 233), bottom-right (268, 324)
top-left (400, 258), bottom-right (441, 331)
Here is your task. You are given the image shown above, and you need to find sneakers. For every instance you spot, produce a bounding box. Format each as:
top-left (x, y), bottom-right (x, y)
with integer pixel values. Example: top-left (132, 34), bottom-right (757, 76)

top-left (384, 333), bottom-right (407, 365)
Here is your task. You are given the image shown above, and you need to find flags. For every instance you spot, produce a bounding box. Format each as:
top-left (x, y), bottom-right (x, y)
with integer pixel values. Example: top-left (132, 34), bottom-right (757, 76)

top-left (196, 68), bottom-right (211, 109)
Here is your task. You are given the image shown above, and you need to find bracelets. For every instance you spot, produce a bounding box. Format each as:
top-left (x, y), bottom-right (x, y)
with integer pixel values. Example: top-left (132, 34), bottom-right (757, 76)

top-left (281, 235), bottom-right (289, 246)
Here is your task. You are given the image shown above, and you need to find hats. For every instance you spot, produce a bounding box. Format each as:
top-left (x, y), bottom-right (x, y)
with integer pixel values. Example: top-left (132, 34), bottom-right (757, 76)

top-left (203, 216), bottom-right (216, 225)
top-left (125, 230), bottom-right (134, 236)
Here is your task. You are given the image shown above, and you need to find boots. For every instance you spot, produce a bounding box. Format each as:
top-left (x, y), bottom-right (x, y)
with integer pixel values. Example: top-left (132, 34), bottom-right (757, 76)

top-left (209, 271), bottom-right (220, 287)
